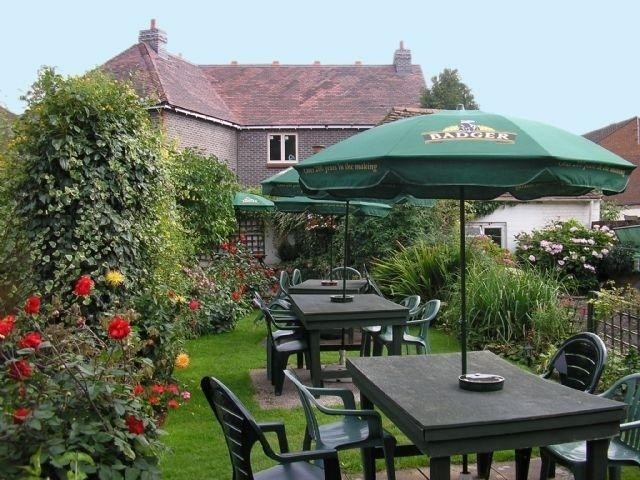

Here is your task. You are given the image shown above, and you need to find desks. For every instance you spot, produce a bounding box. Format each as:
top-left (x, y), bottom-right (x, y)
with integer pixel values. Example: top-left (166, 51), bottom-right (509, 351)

top-left (346, 350), bottom-right (631, 480)
top-left (288, 294), bottom-right (411, 399)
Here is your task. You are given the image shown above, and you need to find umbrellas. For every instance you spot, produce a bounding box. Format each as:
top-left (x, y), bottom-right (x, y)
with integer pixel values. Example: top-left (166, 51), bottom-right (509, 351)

top-left (231, 189), bottom-right (277, 212)
top-left (292, 101), bottom-right (637, 383)
top-left (260, 161), bottom-right (440, 300)
top-left (273, 192), bottom-right (393, 285)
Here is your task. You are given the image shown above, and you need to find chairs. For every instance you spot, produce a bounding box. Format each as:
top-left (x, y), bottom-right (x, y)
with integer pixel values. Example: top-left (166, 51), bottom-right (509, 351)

top-left (252, 266), bottom-right (441, 396)
top-left (516, 332), bottom-right (640, 480)
top-left (200, 369), bottom-right (397, 480)
